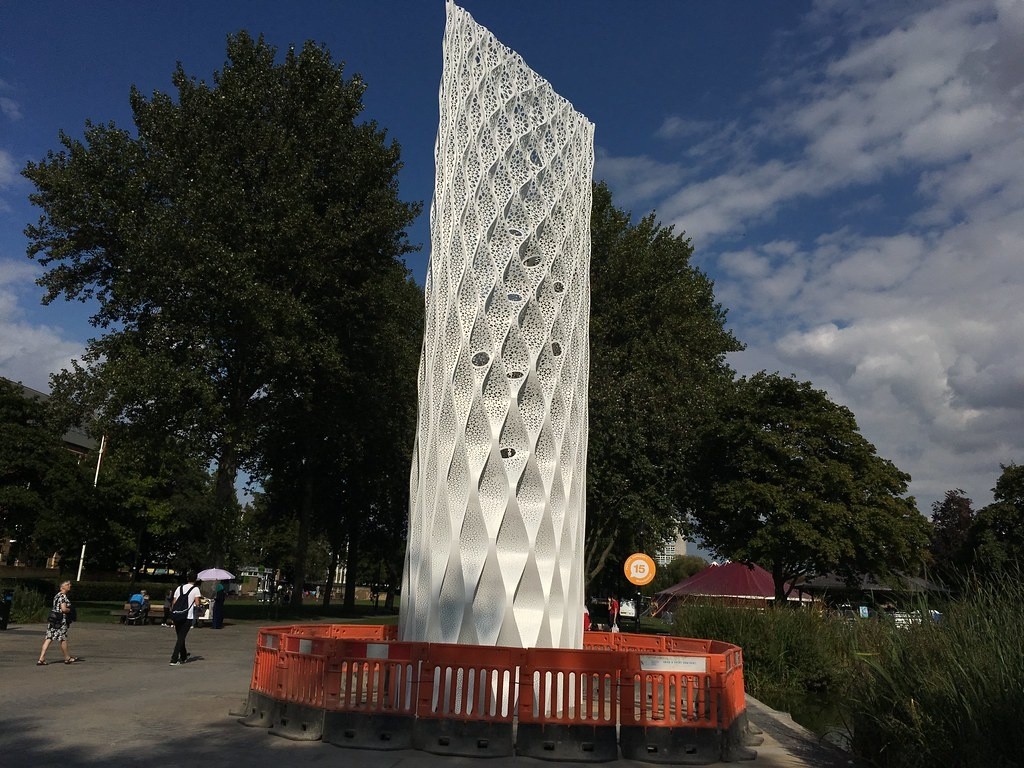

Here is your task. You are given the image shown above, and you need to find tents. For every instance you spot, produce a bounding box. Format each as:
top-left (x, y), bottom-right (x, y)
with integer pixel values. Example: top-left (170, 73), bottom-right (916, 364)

top-left (792, 555), bottom-right (955, 619)
top-left (650, 557), bottom-right (822, 625)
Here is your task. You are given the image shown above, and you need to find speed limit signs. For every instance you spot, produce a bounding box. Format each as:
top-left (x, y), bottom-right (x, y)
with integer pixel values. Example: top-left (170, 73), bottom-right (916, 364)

top-left (624, 553), bottom-right (656, 586)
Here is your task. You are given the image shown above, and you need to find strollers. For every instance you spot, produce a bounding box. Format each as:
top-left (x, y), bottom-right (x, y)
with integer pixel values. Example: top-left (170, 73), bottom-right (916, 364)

top-left (123, 593), bottom-right (150, 626)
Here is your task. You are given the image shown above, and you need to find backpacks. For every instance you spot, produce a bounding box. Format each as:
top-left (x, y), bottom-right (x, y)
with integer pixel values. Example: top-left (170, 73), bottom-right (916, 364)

top-left (170, 583), bottom-right (197, 622)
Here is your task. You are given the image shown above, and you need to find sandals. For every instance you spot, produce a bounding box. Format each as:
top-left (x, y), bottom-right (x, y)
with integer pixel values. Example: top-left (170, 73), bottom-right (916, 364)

top-left (64, 656), bottom-right (77, 664)
top-left (37, 659), bottom-right (48, 664)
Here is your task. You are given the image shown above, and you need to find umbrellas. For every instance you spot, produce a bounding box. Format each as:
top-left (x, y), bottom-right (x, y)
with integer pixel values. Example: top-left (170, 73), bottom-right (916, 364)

top-left (196, 568), bottom-right (235, 580)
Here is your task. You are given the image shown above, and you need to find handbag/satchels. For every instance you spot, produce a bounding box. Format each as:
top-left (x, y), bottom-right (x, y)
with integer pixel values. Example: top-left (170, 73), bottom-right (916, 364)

top-left (611, 624), bottom-right (619, 633)
top-left (48, 611), bottom-right (63, 629)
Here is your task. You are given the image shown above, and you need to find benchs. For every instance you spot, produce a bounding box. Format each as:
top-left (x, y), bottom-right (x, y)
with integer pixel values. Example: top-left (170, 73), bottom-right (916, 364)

top-left (110, 604), bottom-right (171, 624)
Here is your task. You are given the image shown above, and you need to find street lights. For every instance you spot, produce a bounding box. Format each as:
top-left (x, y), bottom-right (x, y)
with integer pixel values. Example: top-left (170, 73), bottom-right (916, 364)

top-left (635, 518), bottom-right (645, 634)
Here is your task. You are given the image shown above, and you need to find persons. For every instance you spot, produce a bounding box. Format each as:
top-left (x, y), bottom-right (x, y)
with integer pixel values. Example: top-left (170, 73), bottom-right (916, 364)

top-left (584, 595), bottom-right (620, 634)
top-left (161, 589), bottom-right (175, 627)
top-left (169, 571), bottom-right (202, 666)
top-left (37, 577), bottom-right (79, 665)
top-left (140, 590), bottom-right (150, 624)
top-left (220, 579), bottom-right (378, 606)
top-left (210, 583), bottom-right (226, 629)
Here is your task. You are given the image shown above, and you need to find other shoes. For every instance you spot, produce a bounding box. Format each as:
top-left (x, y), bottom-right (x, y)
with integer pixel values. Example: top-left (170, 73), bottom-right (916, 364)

top-left (169, 660), bottom-right (180, 665)
top-left (211, 627), bottom-right (216, 629)
top-left (161, 624), bottom-right (167, 626)
top-left (181, 653), bottom-right (190, 663)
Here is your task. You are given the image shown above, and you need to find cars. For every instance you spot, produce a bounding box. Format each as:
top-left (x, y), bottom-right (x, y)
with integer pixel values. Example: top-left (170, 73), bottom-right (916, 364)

top-left (639, 597), bottom-right (651, 614)
top-left (618, 601), bottom-right (637, 620)
top-left (587, 598), bottom-right (609, 617)
top-left (821, 599), bottom-right (895, 624)
